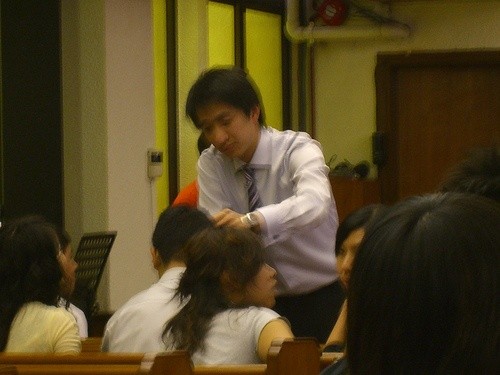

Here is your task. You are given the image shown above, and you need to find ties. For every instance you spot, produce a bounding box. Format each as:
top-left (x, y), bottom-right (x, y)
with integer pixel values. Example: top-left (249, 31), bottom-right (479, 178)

top-left (240, 165), bottom-right (262, 213)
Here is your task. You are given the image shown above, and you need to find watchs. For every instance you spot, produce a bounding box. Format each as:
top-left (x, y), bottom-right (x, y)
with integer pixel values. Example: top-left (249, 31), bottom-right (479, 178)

top-left (241, 211), bottom-right (263, 235)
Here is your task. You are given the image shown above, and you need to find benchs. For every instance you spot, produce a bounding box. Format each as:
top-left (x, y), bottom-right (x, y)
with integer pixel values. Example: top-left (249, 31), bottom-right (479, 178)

top-left (0, 337), bottom-right (322, 375)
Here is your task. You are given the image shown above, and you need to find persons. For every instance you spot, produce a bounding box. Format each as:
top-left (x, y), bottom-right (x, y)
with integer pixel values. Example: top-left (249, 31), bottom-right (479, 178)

top-left (344, 192), bottom-right (500, 374)
top-left (185, 67), bottom-right (344, 345)
top-left (100, 203), bottom-right (217, 352)
top-left (171, 131), bottom-right (212, 207)
top-left (1, 216), bottom-right (83, 354)
top-left (161, 225), bottom-right (295, 365)
top-left (56, 227), bottom-right (88, 339)
top-left (323, 204), bottom-right (383, 343)
